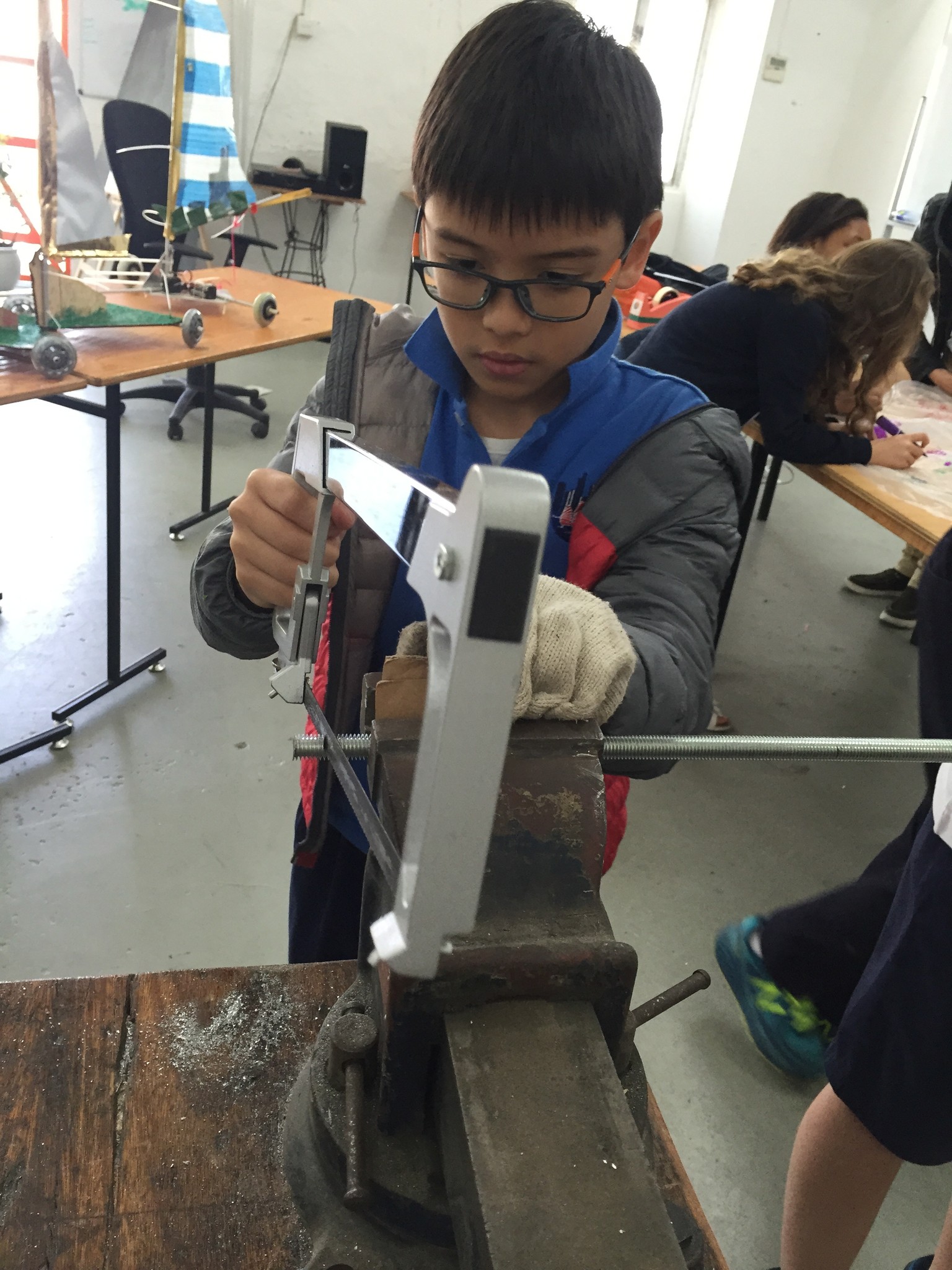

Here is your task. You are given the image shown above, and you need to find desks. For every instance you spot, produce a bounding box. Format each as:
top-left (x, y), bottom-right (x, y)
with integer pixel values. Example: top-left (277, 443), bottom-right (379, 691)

top-left (0, 185), bottom-right (397, 764)
top-left (711, 352), bottom-right (952, 666)
top-left (0, 955), bottom-right (721, 1270)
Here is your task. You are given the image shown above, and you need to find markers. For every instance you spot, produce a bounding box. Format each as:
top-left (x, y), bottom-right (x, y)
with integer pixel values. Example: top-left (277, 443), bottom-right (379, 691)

top-left (876, 415), bottom-right (928, 457)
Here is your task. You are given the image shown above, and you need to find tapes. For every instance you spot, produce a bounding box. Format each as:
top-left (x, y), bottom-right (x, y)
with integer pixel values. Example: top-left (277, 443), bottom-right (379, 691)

top-left (652, 286), bottom-right (681, 306)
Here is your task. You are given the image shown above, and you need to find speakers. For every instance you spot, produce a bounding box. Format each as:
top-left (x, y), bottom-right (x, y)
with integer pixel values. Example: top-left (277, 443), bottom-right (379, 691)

top-left (321, 122), bottom-right (368, 199)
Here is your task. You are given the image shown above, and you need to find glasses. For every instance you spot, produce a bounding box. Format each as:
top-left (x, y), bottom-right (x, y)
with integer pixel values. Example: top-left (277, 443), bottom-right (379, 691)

top-left (412, 198), bottom-right (646, 322)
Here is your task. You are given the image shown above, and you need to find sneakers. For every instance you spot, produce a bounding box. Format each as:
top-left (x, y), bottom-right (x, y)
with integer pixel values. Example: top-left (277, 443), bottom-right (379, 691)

top-left (714, 913), bottom-right (831, 1075)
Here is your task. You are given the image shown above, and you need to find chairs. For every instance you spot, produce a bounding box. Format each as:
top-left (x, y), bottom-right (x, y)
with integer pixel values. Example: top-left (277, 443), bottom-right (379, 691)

top-left (103, 99), bottom-right (297, 438)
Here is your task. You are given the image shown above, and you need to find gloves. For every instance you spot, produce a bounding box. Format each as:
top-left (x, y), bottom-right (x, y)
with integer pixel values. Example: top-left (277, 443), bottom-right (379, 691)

top-left (397, 574), bottom-right (637, 726)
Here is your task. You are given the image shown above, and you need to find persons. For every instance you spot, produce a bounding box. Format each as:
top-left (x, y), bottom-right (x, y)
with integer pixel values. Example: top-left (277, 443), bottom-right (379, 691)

top-left (716, 527), bottom-right (952, 1270)
top-left (611, 190), bottom-right (871, 362)
top-left (842, 184), bottom-right (952, 627)
top-left (634, 239), bottom-right (934, 731)
top-left (191, 0), bottom-right (750, 967)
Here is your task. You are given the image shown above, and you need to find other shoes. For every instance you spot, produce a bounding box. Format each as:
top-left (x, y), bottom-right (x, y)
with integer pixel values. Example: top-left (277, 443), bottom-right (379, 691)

top-left (846, 568), bottom-right (911, 596)
top-left (706, 702), bottom-right (732, 730)
top-left (879, 586), bottom-right (918, 628)
